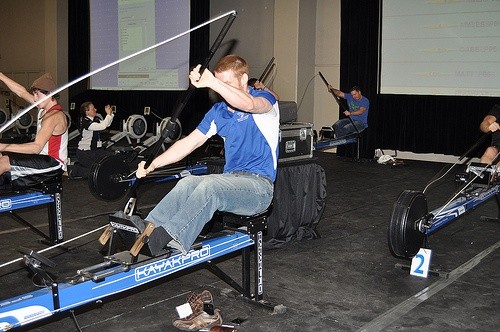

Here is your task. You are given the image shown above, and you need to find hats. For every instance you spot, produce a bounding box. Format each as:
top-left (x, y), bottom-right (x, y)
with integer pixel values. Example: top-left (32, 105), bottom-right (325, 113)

top-left (31, 73), bottom-right (56, 93)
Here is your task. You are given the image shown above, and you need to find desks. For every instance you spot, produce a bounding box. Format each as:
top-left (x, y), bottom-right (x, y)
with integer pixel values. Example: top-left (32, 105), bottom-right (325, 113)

top-left (198, 157), bottom-right (327, 248)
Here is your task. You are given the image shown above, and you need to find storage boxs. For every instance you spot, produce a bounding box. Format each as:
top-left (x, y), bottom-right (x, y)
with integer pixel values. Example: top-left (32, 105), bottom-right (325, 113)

top-left (277, 122), bottom-right (314, 162)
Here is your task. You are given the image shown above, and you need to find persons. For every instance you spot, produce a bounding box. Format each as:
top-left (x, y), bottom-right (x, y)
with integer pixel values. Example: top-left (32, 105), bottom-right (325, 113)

top-left (0, 73), bottom-right (68, 193)
top-left (78, 101), bottom-right (114, 166)
top-left (327, 84), bottom-right (369, 138)
top-left (137, 54), bottom-right (280, 255)
top-left (480, 105), bottom-right (500, 179)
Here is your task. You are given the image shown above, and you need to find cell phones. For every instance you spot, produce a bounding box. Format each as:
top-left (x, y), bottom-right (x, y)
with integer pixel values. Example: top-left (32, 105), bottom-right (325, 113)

top-left (203, 303), bottom-right (214, 316)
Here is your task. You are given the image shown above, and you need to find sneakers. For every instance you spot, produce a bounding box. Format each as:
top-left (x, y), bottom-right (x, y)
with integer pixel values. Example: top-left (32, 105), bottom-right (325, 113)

top-left (184, 289), bottom-right (215, 318)
top-left (171, 308), bottom-right (224, 332)
